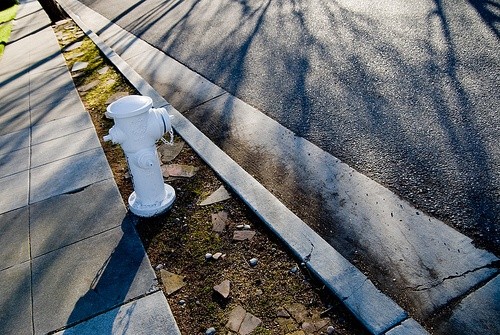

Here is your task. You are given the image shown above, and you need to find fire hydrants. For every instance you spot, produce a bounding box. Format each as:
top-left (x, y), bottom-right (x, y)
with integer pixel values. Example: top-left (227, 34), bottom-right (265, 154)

top-left (102, 94), bottom-right (176, 217)
